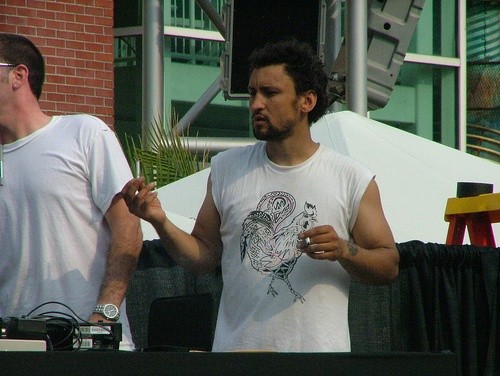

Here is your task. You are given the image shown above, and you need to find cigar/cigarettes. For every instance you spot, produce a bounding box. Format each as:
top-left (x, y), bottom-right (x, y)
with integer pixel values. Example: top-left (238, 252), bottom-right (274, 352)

top-left (136, 159), bottom-right (141, 179)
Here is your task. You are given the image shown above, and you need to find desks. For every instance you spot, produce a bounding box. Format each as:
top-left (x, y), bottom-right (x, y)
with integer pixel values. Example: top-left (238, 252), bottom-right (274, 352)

top-left (0, 351), bottom-right (500, 376)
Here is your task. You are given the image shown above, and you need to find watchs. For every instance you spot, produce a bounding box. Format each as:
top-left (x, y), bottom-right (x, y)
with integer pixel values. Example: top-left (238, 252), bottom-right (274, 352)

top-left (90, 303), bottom-right (121, 323)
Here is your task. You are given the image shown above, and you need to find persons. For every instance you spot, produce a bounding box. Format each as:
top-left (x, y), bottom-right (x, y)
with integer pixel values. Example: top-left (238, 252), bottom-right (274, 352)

top-left (0, 32), bottom-right (144, 323)
top-left (120, 38), bottom-right (401, 353)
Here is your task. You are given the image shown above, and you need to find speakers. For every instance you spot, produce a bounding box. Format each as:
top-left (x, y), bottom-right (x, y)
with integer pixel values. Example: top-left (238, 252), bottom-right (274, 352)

top-left (222, 0), bottom-right (327, 100)
top-left (329, 0), bottom-right (426, 110)
